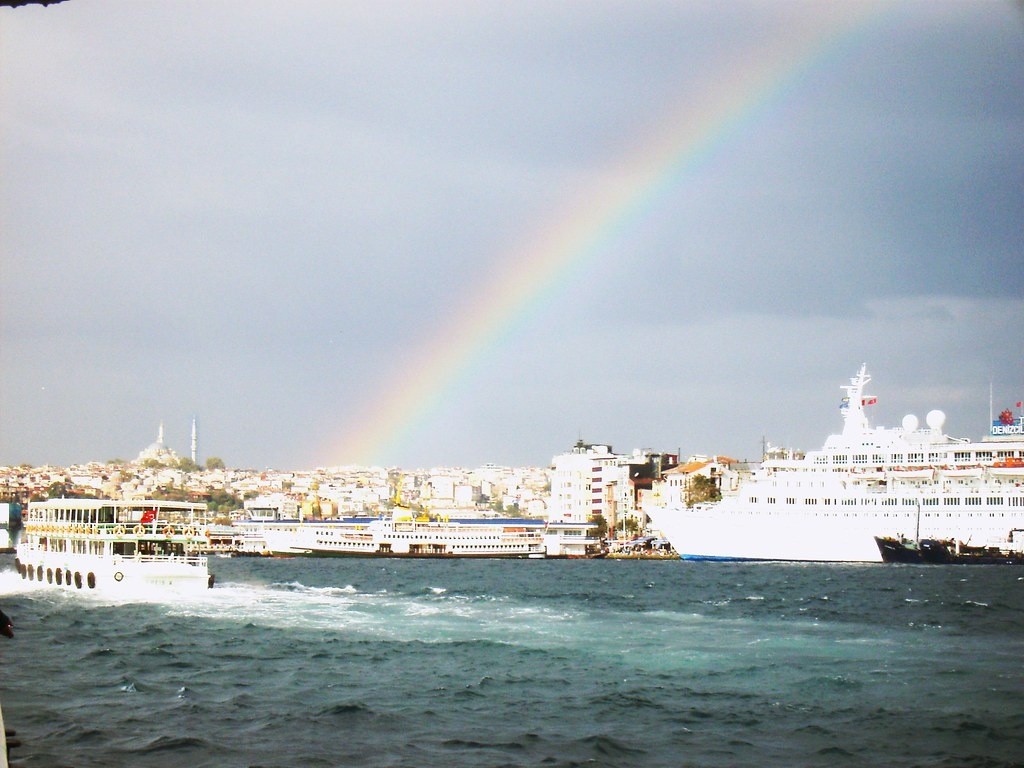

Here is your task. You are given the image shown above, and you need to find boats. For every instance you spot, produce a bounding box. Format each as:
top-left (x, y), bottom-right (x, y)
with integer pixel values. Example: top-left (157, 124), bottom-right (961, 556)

top-left (236, 516), bottom-right (596, 560)
top-left (874, 504), bottom-right (1023, 565)
top-left (14, 497), bottom-right (216, 591)
top-left (640, 361), bottom-right (1023, 563)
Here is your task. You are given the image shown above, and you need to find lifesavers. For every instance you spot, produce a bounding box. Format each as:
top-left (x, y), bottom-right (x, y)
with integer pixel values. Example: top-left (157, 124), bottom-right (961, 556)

top-left (162, 526), bottom-right (175, 538)
top-left (56, 568), bottom-right (62, 586)
top-left (37, 566), bottom-right (43, 581)
top-left (133, 525), bottom-right (145, 537)
top-left (75, 571), bottom-right (82, 589)
top-left (46, 568), bottom-right (53, 584)
top-left (25, 525), bottom-right (100, 538)
top-left (66, 569), bottom-right (71, 586)
top-left (27, 565), bottom-right (34, 580)
top-left (14, 557), bottom-right (26, 579)
top-left (87, 572), bottom-right (95, 588)
top-left (182, 526), bottom-right (194, 539)
top-left (112, 525), bottom-right (125, 539)
